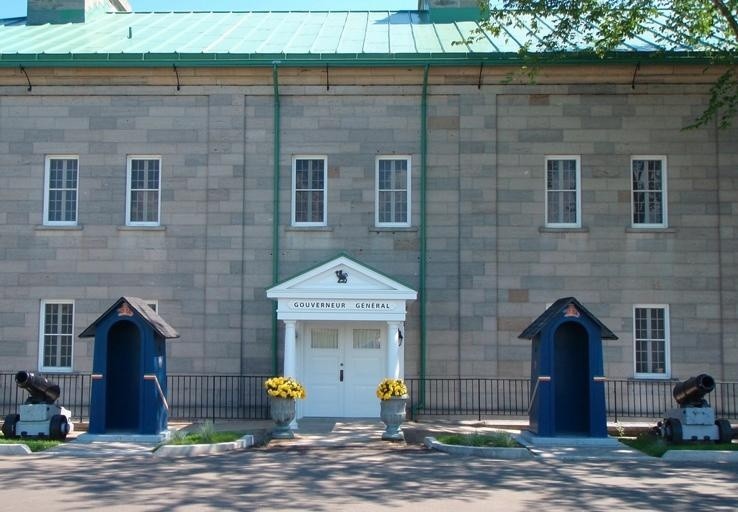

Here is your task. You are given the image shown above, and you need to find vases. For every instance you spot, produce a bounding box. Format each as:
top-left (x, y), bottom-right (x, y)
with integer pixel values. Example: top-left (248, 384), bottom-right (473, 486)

top-left (380, 394), bottom-right (409, 440)
top-left (269, 400), bottom-right (295, 439)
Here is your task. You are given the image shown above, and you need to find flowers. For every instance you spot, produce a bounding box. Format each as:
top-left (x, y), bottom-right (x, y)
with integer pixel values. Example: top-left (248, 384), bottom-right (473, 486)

top-left (265, 376), bottom-right (306, 400)
top-left (376, 379), bottom-right (407, 400)
